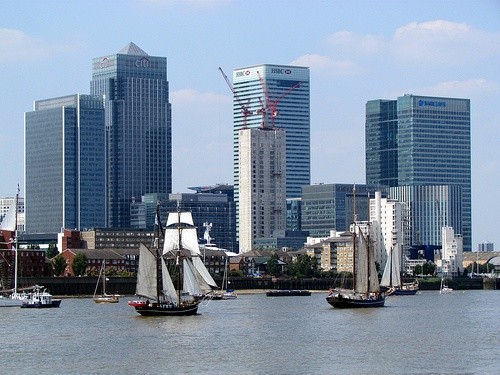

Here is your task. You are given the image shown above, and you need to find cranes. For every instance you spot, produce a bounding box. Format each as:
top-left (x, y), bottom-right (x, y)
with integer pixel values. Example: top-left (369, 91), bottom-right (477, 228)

top-left (218, 68), bottom-right (256, 130)
top-left (253, 70), bottom-right (299, 128)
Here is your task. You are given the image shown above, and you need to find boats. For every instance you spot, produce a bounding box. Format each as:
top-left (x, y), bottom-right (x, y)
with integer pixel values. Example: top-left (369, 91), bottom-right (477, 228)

top-left (19, 285), bottom-right (62, 308)
top-left (439, 277), bottom-right (454, 295)
top-left (265, 290), bottom-right (312, 297)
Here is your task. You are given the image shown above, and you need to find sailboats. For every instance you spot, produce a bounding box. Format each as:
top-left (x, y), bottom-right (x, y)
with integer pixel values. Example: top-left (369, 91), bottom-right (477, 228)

top-left (326, 181), bottom-right (386, 308)
top-left (0, 184), bottom-right (53, 307)
top-left (180, 259), bottom-right (238, 302)
top-left (92, 257), bottom-right (121, 304)
top-left (127, 200), bottom-right (219, 316)
top-left (378, 242), bottom-right (419, 295)
